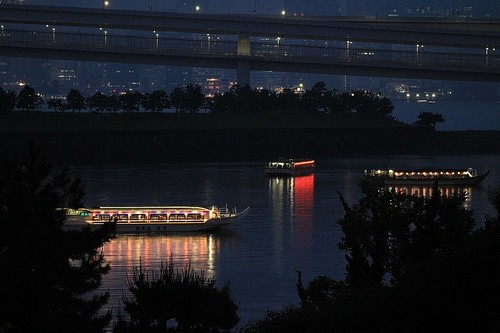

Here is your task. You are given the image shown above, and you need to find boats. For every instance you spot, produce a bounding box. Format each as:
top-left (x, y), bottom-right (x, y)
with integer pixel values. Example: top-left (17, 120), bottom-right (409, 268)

top-left (265, 157), bottom-right (315, 176)
top-left (364, 167), bottom-right (491, 187)
top-left (57, 202), bottom-right (250, 232)
